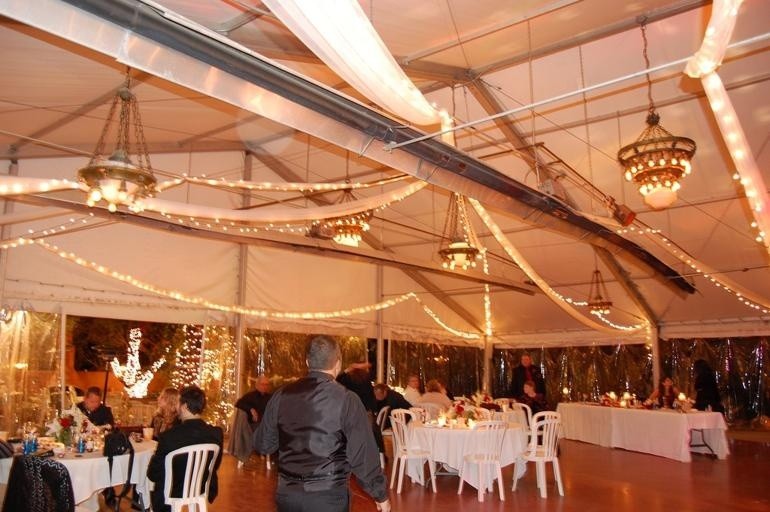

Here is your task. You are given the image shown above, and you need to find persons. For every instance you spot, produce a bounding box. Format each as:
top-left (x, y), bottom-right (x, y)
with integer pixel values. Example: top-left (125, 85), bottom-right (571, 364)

top-left (693, 359), bottom-right (725, 418)
top-left (406, 376), bottom-right (454, 406)
top-left (235, 376), bottom-right (275, 433)
top-left (335, 361), bottom-right (385, 453)
top-left (75, 386), bottom-right (115, 435)
top-left (372, 383), bottom-right (413, 431)
top-left (150, 388), bottom-right (183, 441)
top-left (253, 334), bottom-right (392, 512)
top-left (147, 386), bottom-right (224, 512)
top-left (650, 376), bottom-right (680, 409)
top-left (509, 353), bottom-right (547, 398)
top-left (520, 381), bottom-right (548, 444)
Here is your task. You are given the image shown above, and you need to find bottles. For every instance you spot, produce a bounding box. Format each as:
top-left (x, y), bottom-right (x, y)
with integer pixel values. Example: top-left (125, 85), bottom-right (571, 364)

top-left (79, 438), bottom-right (84, 453)
top-left (421, 410), bottom-right (426, 424)
top-left (31, 438), bottom-right (36, 452)
top-left (24, 439), bottom-right (28, 455)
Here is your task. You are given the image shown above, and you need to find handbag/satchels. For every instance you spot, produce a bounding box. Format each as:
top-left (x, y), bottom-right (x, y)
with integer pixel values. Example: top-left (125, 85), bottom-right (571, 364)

top-left (103, 430), bottom-right (130, 456)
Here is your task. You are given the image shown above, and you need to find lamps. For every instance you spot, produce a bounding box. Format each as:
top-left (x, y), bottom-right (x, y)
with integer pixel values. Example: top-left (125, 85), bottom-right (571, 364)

top-left (78, 68), bottom-right (157, 213)
top-left (587, 246), bottom-right (614, 315)
top-left (316, 150), bottom-right (373, 247)
top-left (617, 14), bottom-right (697, 210)
top-left (435, 191), bottom-right (485, 271)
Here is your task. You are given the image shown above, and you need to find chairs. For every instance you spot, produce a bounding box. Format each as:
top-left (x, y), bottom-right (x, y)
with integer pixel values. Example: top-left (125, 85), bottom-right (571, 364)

top-left (0, 455), bottom-right (75, 511)
top-left (376, 398), bottom-right (564, 504)
top-left (237, 408), bottom-right (271, 470)
top-left (163, 443), bottom-right (221, 511)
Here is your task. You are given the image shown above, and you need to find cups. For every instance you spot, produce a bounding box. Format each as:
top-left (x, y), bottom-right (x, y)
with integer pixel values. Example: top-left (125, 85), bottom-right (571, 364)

top-left (142, 428), bottom-right (154, 441)
top-left (86, 436), bottom-right (93, 452)
